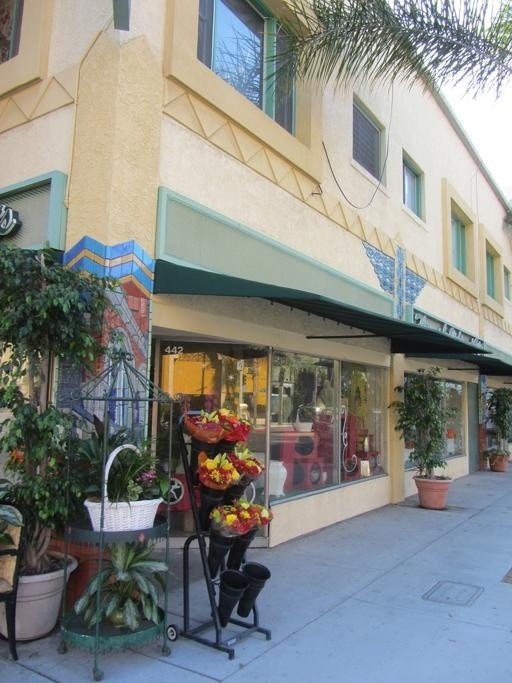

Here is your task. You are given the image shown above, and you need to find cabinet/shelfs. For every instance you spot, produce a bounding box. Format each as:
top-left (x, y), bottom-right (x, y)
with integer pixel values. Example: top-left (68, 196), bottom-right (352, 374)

top-left (54, 350), bottom-right (185, 681)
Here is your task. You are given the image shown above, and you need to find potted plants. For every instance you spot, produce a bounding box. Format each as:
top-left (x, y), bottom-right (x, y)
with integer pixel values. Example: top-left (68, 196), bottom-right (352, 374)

top-left (386, 366), bottom-right (464, 510)
top-left (480, 384), bottom-right (512, 472)
top-left (0, 241), bottom-right (116, 641)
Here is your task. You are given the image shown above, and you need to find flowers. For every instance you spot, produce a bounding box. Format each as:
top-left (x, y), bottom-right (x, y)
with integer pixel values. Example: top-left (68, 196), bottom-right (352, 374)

top-left (188, 406), bottom-right (274, 535)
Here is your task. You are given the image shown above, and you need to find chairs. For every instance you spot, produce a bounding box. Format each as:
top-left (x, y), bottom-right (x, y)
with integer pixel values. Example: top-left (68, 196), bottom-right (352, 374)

top-left (0, 502), bottom-right (26, 662)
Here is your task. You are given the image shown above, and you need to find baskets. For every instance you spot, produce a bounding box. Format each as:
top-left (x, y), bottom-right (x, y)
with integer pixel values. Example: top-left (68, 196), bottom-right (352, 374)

top-left (84, 444), bottom-right (164, 532)
